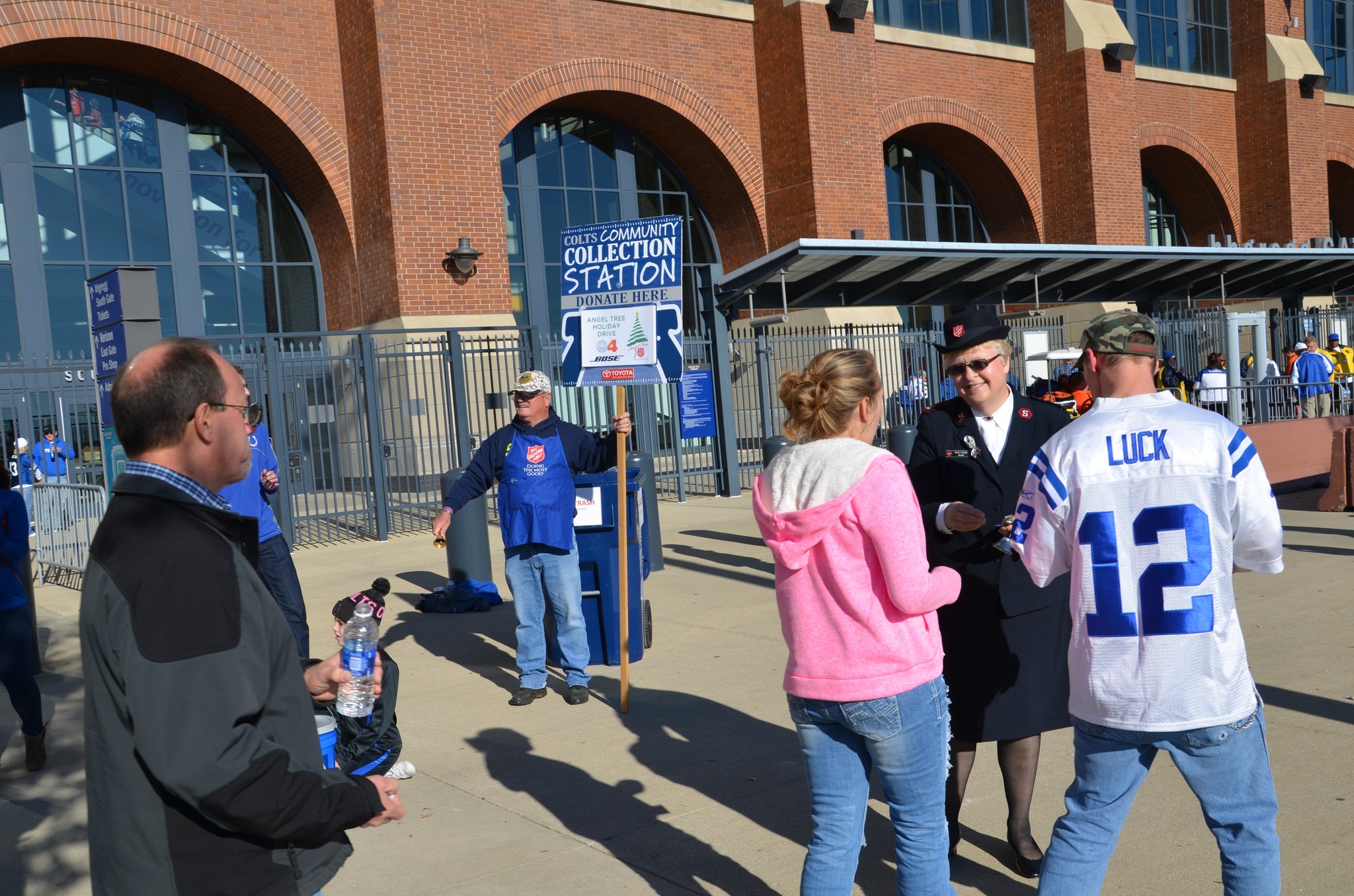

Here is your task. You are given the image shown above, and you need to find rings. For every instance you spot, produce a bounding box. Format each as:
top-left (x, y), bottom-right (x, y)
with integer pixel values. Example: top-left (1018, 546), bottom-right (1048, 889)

top-left (627, 423), bottom-right (629, 428)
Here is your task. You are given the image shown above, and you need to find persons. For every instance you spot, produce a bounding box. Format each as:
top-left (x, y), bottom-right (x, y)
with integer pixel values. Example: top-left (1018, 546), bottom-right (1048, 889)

top-left (31, 425), bottom-right (75, 534)
top-left (0, 456), bottom-right (46, 772)
top-left (1011, 308), bottom-right (1286, 895)
top-left (907, 310), bottom-right (1071, 878)
top-left (9, 438), bottom-right (44, 537)
top-left (753, 347), bottom-right (962, 896)
top-left (900, 334), bottom-right (1354, 426)
top-left (328, 577), bottom-right (416, 782)
top-left (433, 371), bottom-right (631, 706)
top-left (81, 338), bottom-right (402, 895)
top-left (215, 364), bottom-right (311, 659)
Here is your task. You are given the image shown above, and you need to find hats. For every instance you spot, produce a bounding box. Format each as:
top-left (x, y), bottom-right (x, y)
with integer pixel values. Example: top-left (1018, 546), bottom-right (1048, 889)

top-left (331, 577), bottom-right (391, 628)
top-left (932, 309), bottom-right (1010, 355)
top-left (507, 370), bottom-right (552, 398)
top-left (1328, 333), bottom-right (1340, 340)
top-left (1294, 342), bottom-right (1308, 353)
top-left (1069, 308), bottom-right (1159, 374)
top-left (1162, 350), bottom-right (1176, 360)
top-left (43, 424), bottom-right (57, 432)
top-left (13, 437), bottom-right (33, 448)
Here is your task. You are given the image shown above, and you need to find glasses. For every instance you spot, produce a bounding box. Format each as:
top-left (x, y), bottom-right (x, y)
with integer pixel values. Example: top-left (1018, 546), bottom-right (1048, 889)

top-left (43, 431), bottom-right (52, 435)
top-left (510, 393), bottom-right (546, 401)
top-left (945, 353), bottom-right (1002, 376)
top-left (1170, 357), bottom-right (1177, 360)
top-left (188, 401), bottom-right (264, 428)
top-left (1220, 359), bottom-right (1225, 362)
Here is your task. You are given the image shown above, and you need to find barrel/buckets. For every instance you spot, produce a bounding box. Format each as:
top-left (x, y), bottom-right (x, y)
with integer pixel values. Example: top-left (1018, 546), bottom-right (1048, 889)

top-left (312, 711), bottom-right (342, 773)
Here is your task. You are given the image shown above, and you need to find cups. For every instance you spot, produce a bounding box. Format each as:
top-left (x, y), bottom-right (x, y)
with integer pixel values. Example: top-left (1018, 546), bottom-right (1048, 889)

top-left (433, 586), bottom-right (445, 593)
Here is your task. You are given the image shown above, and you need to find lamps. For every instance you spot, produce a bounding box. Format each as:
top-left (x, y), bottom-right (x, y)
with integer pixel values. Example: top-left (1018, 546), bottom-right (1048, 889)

top-left (1105, 43), bottom-right (1137, 61)
top-left (1301, 73), bottom-right (1331, 89)
top-left (445, 236), bottom-right (484, 273)
top-left (829, 0), bottom-right (869, 19)
top-left (1284, 17), bottom-right (1299, 33)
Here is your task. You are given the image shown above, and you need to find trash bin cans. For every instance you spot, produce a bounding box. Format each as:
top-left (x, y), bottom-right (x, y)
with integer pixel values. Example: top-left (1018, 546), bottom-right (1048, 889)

top-left (544, 468), bottom-right (654, 667)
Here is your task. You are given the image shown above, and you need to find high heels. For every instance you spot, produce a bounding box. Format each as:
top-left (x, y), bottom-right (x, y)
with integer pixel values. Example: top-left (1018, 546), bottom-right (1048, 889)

top-left (947, 824), bottom-right (962, 857)
top-left (1005, 830), bottom-right (1044, 879)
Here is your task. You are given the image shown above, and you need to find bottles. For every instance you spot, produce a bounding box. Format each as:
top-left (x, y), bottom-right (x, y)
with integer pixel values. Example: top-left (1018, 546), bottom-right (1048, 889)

top-left (447, 578), bottom-right (456, 592)
top-left (333, 602), bottom-right (379, 718)
top-left (49, 444), bottom-right (55, 458)
top-left (1342, 386), bottom-right (1351, 400)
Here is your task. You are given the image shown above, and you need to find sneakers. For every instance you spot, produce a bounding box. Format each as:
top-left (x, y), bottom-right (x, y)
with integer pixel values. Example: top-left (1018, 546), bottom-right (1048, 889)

top-left (28, 532), bottom-right (36, 537)
top-left (510, 686), bottom-right (547, 705)
top-left (54, 527), bottom-right (69, 533)
top-left (384, 760), bottom-right (417, 781)
top-left (44, 528), bottom-right (50, 535)
top-left (22, 720), bottom-right (51, 772)
top-left (568, 684), bottom-right (589, 705)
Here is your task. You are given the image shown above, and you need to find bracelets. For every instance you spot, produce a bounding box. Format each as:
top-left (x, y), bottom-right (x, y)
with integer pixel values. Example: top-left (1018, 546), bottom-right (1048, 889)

top-left (440, 507), bottom-right (453, 517)
